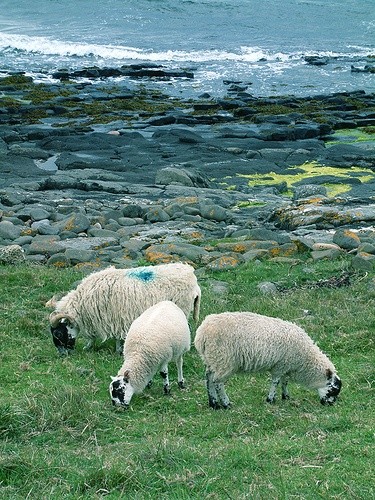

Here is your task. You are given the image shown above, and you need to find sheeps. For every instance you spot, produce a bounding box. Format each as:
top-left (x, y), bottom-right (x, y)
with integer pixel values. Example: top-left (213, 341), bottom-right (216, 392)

top-left (49, 261), bottom-right (202, 356)
top-left (194, 311), bottom-right (342, 410)
top-left (108, 300), bottom-right (191, 413)
